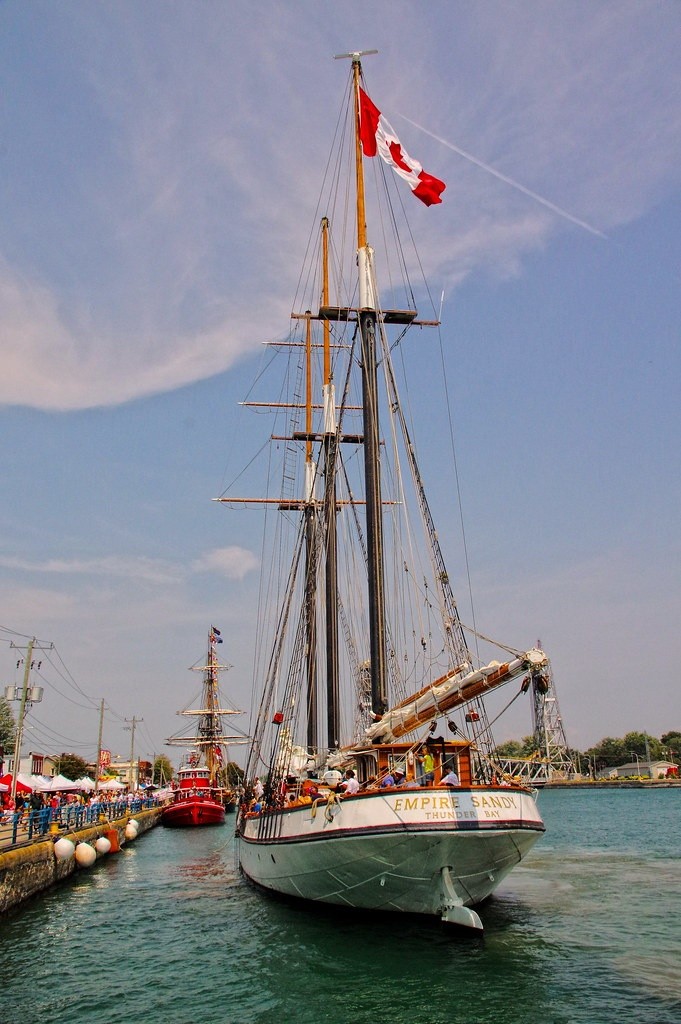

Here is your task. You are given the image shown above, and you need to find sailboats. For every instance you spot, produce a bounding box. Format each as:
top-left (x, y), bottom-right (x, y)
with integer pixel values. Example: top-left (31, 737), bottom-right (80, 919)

top-left (161, 625), bottom-right (259, 812)
top-left (212, 48), bottom-right (551, 930)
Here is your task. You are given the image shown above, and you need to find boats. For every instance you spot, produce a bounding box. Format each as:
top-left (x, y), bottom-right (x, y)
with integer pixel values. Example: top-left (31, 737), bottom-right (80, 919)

top-left (162, 751), bottom-right (226, 826)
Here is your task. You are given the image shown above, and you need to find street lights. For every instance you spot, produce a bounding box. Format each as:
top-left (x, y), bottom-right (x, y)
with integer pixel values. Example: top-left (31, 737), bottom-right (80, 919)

top-left (631, 753), bottom-right (640, 777)
top-left (584, 758), bottom-right (591, 779)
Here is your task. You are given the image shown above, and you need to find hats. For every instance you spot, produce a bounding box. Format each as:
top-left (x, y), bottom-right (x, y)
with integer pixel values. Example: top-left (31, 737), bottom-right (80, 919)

top-left (17, 792), bottom-right (20, 794)
top-left (513, 775), bottom-right (521, 781)
top-left (379, 766), bottom-right (388, 772)
top-left (251, 799), bottom-right (256, 804)
top-left (394, 768), bottom-right (404, 775)
top-left (35, 790), bottom-right (41, 794)
top-left (339, 781), bottom-right (348, 786)
top-left (23, 796), bottom-right (29, 798)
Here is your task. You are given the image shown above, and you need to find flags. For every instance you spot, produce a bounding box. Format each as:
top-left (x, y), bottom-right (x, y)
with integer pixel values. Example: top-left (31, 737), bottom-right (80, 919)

top-left (355, 83), bottom-right (446, 207)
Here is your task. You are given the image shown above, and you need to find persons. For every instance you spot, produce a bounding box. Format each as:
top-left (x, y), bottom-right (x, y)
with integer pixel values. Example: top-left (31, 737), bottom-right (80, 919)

top-left (0, 788), bottom-right (153, 844)
top-left (241, 765), bottom-right (421, 814)
top-left (498, 772), bottom-right (534, 792)
top-left (414, 747), bottom-right (436, 786)
top-left (435, 764), bottom-right (461, 786)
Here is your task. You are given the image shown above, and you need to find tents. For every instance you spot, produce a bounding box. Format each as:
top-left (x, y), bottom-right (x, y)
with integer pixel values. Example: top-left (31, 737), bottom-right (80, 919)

top-left (0, 771), bottom-right (160, 823)
